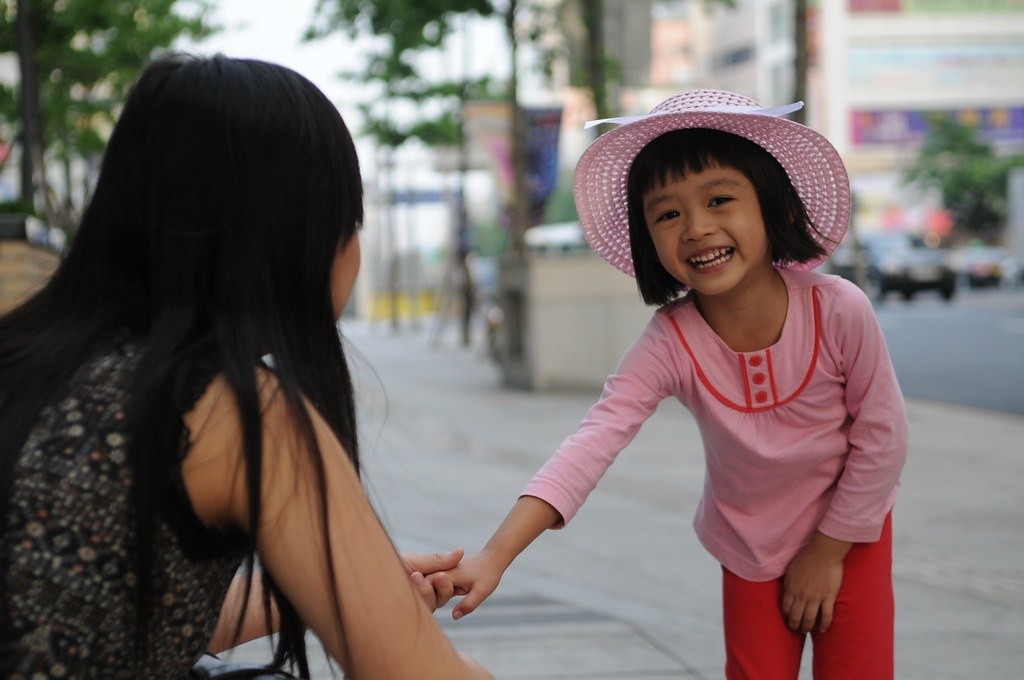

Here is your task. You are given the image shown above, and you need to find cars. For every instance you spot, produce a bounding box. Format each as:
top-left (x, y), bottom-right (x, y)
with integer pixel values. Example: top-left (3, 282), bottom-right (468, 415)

top-left (861, 228), bottom-right (1012, 302)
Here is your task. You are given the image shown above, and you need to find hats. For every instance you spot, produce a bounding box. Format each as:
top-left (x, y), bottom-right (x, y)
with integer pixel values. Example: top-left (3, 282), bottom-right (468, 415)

top-left (572, 90), bottom-right (853, 291)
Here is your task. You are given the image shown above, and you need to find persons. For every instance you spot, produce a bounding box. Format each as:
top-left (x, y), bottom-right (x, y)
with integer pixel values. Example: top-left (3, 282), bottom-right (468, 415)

top-left (423, 87), bottom-right (910, 680)
top-left (0, 49), bottom-right (498, 680)
